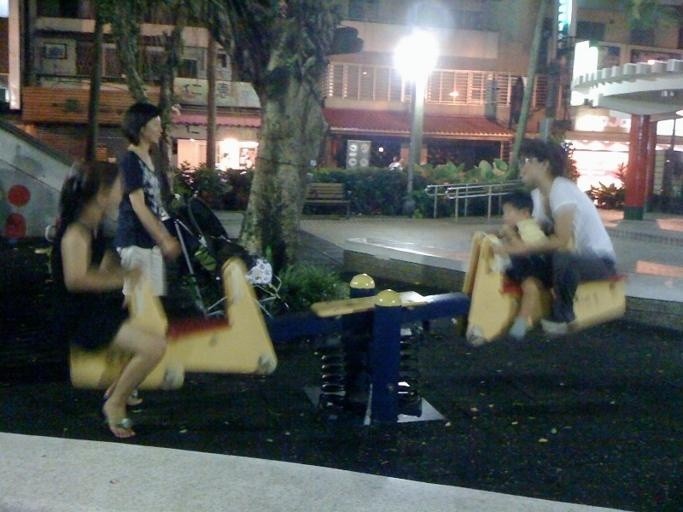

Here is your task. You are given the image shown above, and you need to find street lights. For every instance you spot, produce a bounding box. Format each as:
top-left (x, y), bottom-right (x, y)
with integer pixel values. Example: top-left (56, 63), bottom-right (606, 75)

top-left (400, 29), bottom-right (443, 210)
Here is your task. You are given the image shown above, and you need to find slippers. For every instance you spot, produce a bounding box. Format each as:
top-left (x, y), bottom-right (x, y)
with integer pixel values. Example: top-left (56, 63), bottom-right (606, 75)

top-left (101, 391), bottom-right (145, 408)
top-left (100, 405), bottom-right (138, 441)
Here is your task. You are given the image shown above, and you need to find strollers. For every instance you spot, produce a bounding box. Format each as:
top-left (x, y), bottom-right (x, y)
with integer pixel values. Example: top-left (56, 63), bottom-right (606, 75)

top-left (164, 191), bottom-right (313, 386)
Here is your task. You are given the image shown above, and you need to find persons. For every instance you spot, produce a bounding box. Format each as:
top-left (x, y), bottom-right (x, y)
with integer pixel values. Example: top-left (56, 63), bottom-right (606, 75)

top-left (387, 155), bottom-right (403, 171)
top-left (110, 100), bottom-right (183, 301)
top-left (482, 134), bottom-right (619, 341)
top-left (46, 158), bottom-right (173, 439)
top-left (500, 186), bottom-right (554, 344)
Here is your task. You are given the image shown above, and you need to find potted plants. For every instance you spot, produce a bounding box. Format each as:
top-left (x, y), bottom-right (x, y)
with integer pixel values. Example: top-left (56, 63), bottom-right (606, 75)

top-left (194, 164), bottom-right (254, 209)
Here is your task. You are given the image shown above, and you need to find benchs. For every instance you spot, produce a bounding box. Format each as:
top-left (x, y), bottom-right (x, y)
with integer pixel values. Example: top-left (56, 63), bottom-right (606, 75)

top-left (304, 183), bottom-right (352, 219)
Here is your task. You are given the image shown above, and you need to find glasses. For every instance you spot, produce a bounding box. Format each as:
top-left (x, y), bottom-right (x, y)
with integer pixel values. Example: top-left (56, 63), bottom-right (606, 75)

top-left (519, 156), bottom-right (537, 169)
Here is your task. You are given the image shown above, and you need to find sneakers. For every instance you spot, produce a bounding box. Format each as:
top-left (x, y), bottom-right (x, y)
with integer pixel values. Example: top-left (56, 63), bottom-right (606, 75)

top-left (541, 309), bottom-right (578, 328)
